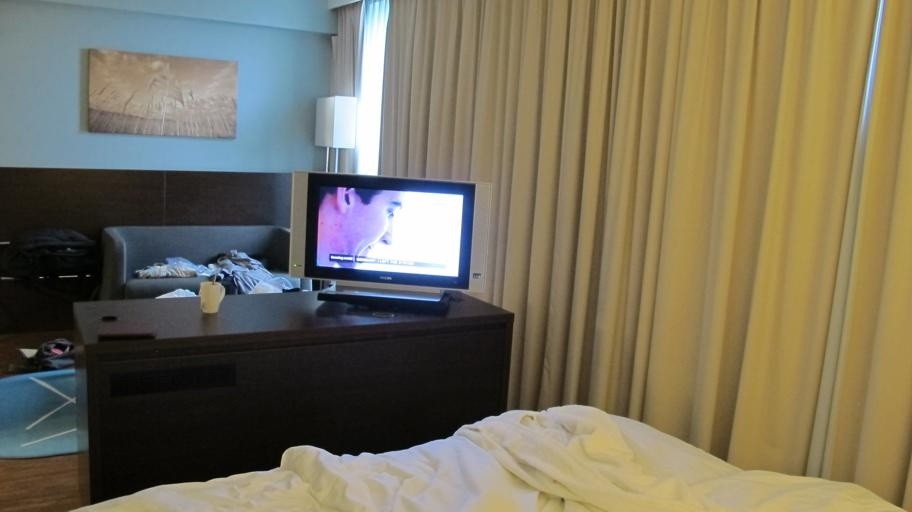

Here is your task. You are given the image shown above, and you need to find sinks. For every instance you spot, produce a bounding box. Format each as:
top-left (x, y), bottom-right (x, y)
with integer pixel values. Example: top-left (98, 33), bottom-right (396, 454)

top-left (99, 225), bottom-right (290, 300)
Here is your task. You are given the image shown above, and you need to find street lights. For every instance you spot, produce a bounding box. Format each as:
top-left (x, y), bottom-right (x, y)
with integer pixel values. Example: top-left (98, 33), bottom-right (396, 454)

top-left (35, 336), bottom-right (75, 370)
top-left (3, 228), bottom-right (102, 278)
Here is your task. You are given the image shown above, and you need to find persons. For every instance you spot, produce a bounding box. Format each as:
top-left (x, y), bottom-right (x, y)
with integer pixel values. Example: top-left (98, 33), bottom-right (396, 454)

top-left (316, 188), bottom-right (406, 267)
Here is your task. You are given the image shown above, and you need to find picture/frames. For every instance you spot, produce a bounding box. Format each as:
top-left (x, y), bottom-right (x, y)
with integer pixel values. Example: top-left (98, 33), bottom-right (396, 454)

top-left (75, 295), bottom-right (514, 507)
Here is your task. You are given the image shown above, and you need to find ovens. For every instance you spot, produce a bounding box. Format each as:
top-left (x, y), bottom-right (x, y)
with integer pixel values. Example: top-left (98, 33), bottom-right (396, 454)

top-left (72, 404), bottom-right (910, 511)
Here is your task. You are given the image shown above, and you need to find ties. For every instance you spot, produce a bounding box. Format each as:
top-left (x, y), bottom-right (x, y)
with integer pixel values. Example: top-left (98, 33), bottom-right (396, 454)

top-left (287, 169), bottom-right (494, 311)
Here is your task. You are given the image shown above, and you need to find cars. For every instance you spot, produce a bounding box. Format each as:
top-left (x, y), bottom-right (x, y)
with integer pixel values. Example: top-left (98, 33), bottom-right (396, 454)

top-left (200, 281), bottom-right (225, 314)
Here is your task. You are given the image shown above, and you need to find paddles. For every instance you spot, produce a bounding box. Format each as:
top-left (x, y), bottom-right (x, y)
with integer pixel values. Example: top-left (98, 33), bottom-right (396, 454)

top-left (0, 369), bottom-right (79, 460)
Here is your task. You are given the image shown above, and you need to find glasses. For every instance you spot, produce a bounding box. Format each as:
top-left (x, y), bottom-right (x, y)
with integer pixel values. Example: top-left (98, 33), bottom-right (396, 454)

top-left (315, 96), bottom-right (357, 174)
